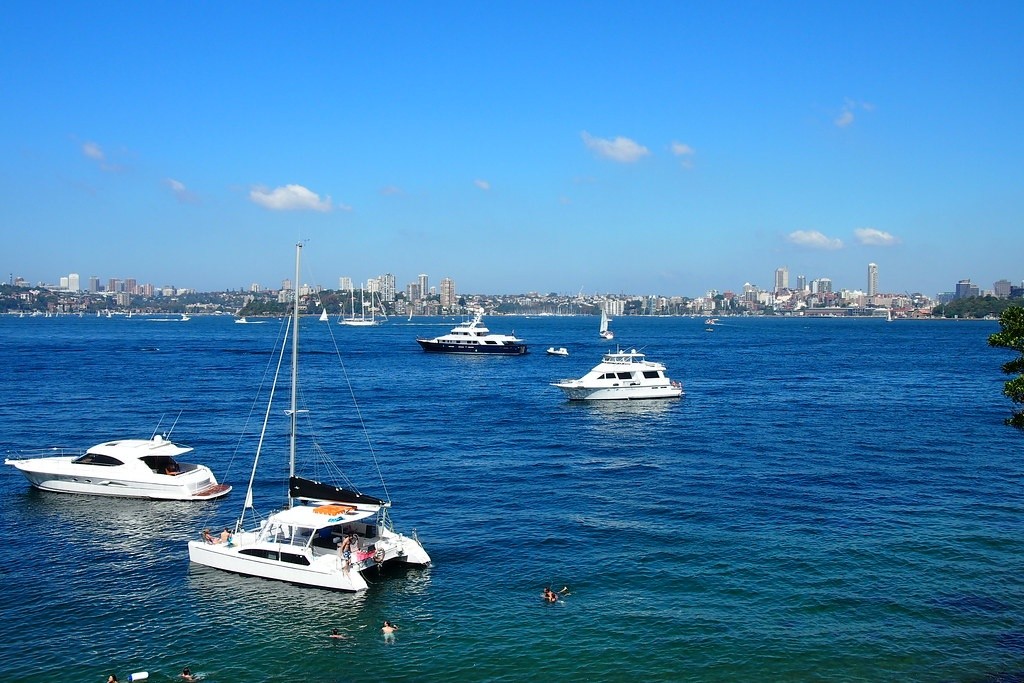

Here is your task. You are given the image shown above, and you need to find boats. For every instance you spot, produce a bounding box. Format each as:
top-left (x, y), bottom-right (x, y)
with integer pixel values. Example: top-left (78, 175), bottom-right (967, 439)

top-left (180, 314), bottom-right (191, 322)
top-left (4, 407), bottom-right (233, 501)
top-left (548, 345), bottom-right (687, 400)
top-left (234, 315), bottom-right (268, 324)
top-left (416, 309), bottom-right (529, 355)
top-left (547, 347), bottom-right (569, 356)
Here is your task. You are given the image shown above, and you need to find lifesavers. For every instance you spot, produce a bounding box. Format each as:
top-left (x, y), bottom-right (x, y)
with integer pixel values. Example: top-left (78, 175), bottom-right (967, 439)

top-left (373, 548), bottom-right (385, 563)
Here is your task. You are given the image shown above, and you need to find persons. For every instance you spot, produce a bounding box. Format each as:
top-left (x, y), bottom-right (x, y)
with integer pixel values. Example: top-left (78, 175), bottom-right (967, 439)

top-left (330, 628), bottom-right (343, 639)
top-left (381, 620), bottom-right (399, 644)
top-left (205, 527), bottom-right (230, 544)
top-left (543, 587), bottom-right (559, 603)
top-left (672, 380), bottom-right (682, 388)
top-left (340, 533), bottom-right (353, 572)
top-left (107, 674), bottom-right (119, 683)
top-left (181, 667), bottom-right (200, 683)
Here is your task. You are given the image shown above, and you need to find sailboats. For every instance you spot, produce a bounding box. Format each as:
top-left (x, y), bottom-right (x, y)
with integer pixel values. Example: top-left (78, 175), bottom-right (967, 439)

top-left (18, 308), bottom-right (133, 318)
top-left (598, 308), bottom-right (614, 339)
top-left (407, 309), bottom-right (413, 322)
top-left (186, 242), bottom-right (432, 593)
top-left (885, 311), bottom-right (894, 321)
top-left (318, 308), bottom-right (329, 322)
top-left (336, 281), bottom-right (389, 326)
